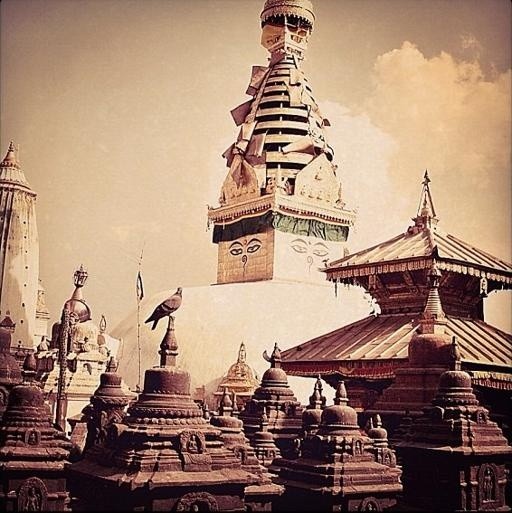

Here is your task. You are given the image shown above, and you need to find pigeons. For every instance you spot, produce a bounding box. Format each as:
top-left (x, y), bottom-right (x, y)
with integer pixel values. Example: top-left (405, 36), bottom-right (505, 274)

top-left (143, 287), bottom-right (183, 331)
